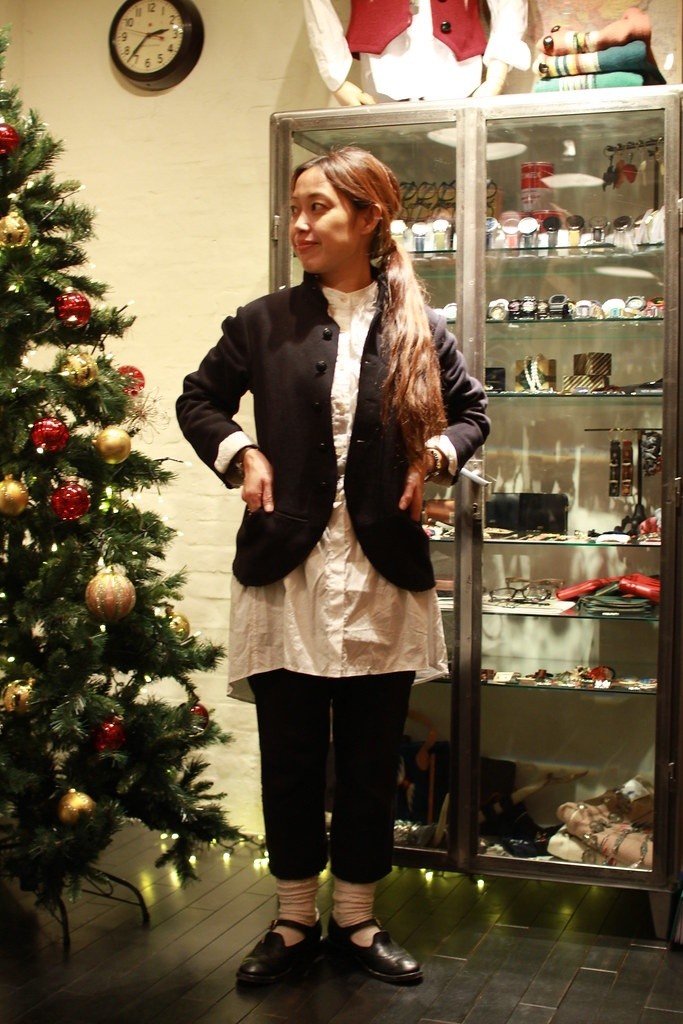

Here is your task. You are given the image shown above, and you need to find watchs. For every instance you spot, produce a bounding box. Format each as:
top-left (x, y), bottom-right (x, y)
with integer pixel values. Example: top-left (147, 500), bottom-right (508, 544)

top-left (435, 292), bottom-right (669, 321)
top-left (383, 209), bottom-right (668, 257)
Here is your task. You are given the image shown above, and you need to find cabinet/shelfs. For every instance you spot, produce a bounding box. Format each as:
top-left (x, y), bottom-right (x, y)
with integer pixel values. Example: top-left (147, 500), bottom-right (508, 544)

top-left (267, 85), bottom-right (683, 952)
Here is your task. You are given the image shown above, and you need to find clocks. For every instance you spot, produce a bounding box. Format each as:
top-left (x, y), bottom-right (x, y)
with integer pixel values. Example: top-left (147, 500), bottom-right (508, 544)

top-left (108, 0), bottom-right (204, 91)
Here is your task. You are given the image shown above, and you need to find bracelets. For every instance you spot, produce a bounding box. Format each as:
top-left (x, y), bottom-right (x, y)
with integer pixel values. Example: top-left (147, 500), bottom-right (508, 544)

top-left (234, 445), bottom-right (254, 477)
top-left (421, 447), bottom-right (444, 480)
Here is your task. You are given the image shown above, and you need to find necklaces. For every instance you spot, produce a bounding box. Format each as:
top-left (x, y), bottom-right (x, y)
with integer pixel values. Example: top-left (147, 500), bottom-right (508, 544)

top-left (522, 351), bottom-right (548, 393)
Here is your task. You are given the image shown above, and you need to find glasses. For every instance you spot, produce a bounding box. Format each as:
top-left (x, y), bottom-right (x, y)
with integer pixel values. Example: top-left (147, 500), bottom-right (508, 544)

top-left (505, 578), bottom-right (563, 599)
top-left (395, 179), bottom-right (497, 223)
top-left (490, 584), bottom-right (552, 602)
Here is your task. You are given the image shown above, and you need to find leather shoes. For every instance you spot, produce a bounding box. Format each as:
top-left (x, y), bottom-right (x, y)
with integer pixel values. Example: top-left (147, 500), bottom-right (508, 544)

top-left (327, 911), bottom-right (423, 983)
top-left (237, 918), bottom-right (323, 986)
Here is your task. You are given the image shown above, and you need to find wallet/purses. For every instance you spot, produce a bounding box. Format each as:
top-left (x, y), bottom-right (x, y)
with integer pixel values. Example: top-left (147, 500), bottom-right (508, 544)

top-left (555, 574), bottom-right (661, 614)
top-left (423, 499), bottom-right (457, 525)
top-left (485, 492), bottom-right (568, 534)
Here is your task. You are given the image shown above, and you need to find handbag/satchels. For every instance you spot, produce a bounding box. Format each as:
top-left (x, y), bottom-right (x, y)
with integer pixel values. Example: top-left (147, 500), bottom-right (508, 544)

top-left (393, 711), bottom-right (449, 827)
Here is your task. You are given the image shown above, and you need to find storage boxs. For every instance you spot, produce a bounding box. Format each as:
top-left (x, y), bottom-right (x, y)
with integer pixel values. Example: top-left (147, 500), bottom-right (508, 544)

top-left (515, 358), bottom-right (557, 392)
top-left (561, 374), bottom-right (610, 392)
top-left (485, 492), bottom-right (569, 534)
top-left (573, 352), bottom-right (613, 377)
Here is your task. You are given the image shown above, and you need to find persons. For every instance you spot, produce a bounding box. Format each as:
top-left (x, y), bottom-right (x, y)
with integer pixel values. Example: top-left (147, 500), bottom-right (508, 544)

top-left (301, 0), bottom-right (535, 109)
top-left (174, 144), bottom-right (492, 994)
